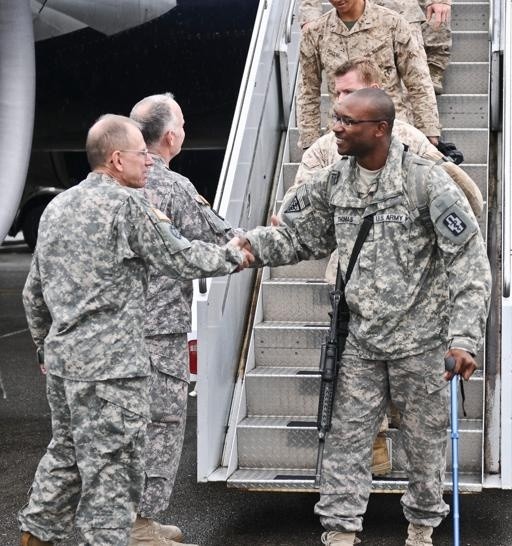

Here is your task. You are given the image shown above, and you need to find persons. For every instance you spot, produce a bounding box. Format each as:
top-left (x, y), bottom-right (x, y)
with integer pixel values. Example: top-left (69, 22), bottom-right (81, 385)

top-left (299, 0), bottom-right (452, 127)
top-left (125, 89), bottom-right (250, 543)
top-left (16, 112), bottom-right (255, 543)
top-left (270, 56), bottom-right (484, 479)
top-left (419, 1), bottom-right (453, 95)
top-left (294, 0), bottom-right (443, 155)
top-left (227, 88), bottom-right (494, 546)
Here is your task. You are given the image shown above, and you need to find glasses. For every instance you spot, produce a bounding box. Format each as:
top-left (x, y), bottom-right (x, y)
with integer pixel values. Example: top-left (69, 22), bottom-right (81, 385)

top-left (332, 112), bottom-right (380, 127)
top-left (111, 149), bottom-right (149, 163)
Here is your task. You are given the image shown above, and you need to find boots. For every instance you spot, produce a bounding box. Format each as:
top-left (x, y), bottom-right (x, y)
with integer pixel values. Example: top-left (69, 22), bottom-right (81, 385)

top-left (405, 523), bottom-right (434, 546)
top-left (430, 65), bottom-right (444, 95)
top-left (321, 531), bottom-right (361, 546)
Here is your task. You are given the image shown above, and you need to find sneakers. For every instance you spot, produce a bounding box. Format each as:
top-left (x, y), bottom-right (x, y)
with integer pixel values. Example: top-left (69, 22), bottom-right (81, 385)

top-left (129, 516), bottom-right (198, 546)
top-left (370, 437), bottom-right (392, 478)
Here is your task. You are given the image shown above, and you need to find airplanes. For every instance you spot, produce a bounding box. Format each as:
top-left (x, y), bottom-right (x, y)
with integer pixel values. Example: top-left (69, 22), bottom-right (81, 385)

top-left (0, 0), bottom-right (509, 497)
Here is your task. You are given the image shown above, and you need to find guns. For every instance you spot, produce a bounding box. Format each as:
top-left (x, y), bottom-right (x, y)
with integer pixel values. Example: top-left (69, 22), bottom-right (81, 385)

top-left (314, 259), bottom-right (350, 490)
